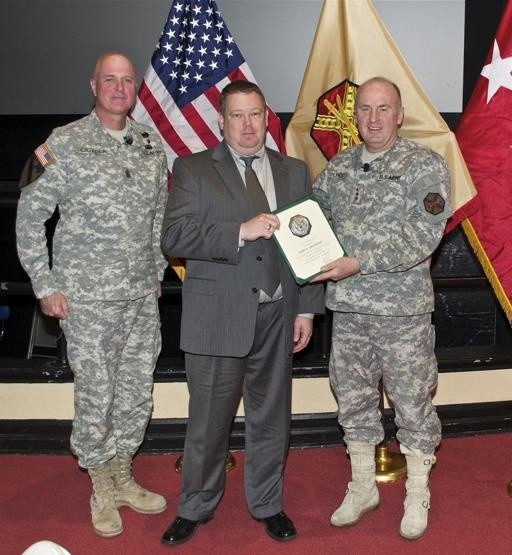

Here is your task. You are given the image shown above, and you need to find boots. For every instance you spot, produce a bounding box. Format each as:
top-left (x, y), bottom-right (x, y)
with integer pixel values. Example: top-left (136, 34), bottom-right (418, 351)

top-left (85, 459), bottom-right (125, 539)
top-left (329, 434), bottom-right (382, 530)
top-left (108, 454), bottom-right (169, 516)
top-left (396, 441), bottom-right (438, 541)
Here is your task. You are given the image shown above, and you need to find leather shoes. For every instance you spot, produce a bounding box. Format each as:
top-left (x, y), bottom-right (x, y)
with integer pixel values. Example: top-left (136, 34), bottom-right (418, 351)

top-left (249, 508), bottom-right (298, 543)
top-left (159, 511), bottom-right (215, 546)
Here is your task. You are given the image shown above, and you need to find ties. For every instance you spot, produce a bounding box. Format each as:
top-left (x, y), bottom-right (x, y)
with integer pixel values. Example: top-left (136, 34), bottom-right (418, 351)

top-left (238, 154), bottom-right (282, 300)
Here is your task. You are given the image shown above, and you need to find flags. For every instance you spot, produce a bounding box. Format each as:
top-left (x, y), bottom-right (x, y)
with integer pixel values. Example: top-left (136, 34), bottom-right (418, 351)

top-left (128, 0), bottom-right (288, 286)
top-left (453, 0), bottom-right (512, 326)
top-left (283, 1), bottom-right (482, 237)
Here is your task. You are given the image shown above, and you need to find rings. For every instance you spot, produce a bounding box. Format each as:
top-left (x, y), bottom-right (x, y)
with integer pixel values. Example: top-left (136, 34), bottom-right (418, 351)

top-left (267, 223), bottom-right (272, 230)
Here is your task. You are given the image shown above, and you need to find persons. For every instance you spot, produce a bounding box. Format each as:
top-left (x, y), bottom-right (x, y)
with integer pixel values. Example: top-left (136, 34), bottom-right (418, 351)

top-left (15, 52), bottom-right (169, 538)
top-left (309, 77), bottom-right (454, 543)
top-left (159, 81), bottom-right (326, 547)
